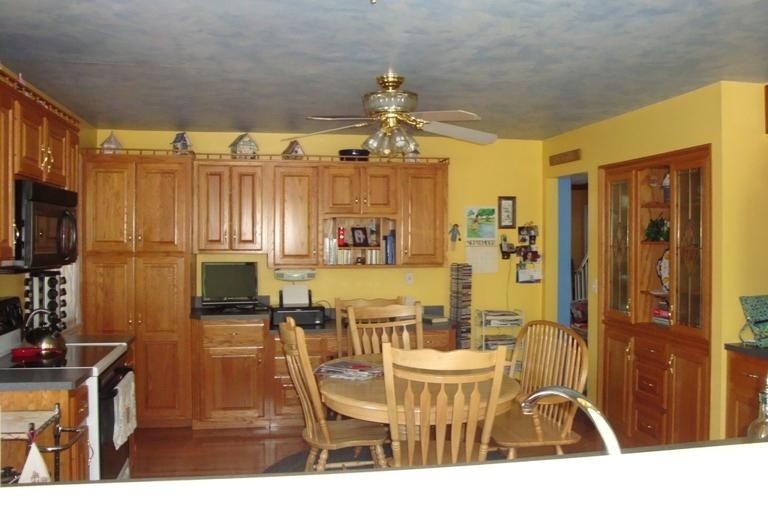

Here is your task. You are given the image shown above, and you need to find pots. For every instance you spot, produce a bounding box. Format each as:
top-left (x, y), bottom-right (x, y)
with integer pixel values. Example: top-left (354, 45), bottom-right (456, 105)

top-left (11, 347), bottom-right (63, 360)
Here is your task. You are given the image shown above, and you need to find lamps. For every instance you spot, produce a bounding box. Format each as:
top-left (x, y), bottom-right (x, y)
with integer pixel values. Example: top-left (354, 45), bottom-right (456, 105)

top-left (359, 117), bottom-right (420, 158)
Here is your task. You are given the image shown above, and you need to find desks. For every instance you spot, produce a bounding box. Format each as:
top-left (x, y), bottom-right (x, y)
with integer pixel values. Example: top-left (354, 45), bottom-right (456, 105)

top-left (314, 352), bottom-right (523, 470)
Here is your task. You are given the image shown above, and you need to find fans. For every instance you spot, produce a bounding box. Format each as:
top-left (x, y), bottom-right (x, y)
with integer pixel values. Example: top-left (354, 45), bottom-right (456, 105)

top-left (278, 72), bottom-right (500, 148)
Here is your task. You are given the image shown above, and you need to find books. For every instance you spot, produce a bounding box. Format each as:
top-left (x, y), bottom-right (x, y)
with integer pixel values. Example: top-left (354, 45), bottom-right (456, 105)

top-left (651, 308), bottom-right (668, 325)
top-left (481, 309), bottom-right (521, 350)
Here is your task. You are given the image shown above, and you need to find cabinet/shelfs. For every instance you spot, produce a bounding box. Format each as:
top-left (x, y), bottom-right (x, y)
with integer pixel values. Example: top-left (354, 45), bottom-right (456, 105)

top-left (324, 166), bottom-right (397, 214)
top-left (83, 155), bottom-right (189, 254)
top-left (69, 386), bottom-right (89, 429)
top-left (275, 167), bottom-right (318, 268)
top-left (598, 316), bottom-right (634, 449)
top-left (14, 101), bottom-right (67, 187)
top-left (133, 334), bottom-right (190, 428)
top-left (401, 165), bottom-right (444, 267)
top-left (668, 141), bottom-right (712, 345)
top-left (631, 322), bottom-right (671, 447)
top-left (195, 318), bottom-right (272, 432)
top-left (630, 151), bottom-right (671, 322)
top-left (596, 159), bottom-right (631, 318)
top-left (722, 343), bottom-right (766, 440)
top-left (82, 254), bottom-right (189, 334)
top-left (67, 128), bottom-right (79, 190)
top-left (667, 338), bottom-right (712, 445)
top-left (398, 332), bottom-right (456, 352)
top-left (470, 308), bottom-right (524, 353)
top-left (0, 105), bottom-right (16, 259)
top-left (199, 165), bottom-right (262, 251)
top-left (322, 213), bottom-right (396, 268)
top-left (275, 334), bottom-right (346, 427)
top-left (72, 429), bottom-right (90, 484)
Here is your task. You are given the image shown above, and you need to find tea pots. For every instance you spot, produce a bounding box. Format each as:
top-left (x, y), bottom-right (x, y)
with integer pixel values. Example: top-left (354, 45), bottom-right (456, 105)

top-left (23, 307), bottom-right (66, 359)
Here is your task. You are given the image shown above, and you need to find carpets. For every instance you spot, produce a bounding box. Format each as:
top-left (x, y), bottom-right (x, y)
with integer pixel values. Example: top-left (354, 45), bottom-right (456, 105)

top-left (262, 441), bottom-right (506, 475)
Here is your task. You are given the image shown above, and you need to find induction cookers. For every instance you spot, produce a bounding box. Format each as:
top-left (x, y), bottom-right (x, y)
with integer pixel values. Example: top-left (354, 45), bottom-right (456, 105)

top-left (0, 342), bottom-right (131, 393)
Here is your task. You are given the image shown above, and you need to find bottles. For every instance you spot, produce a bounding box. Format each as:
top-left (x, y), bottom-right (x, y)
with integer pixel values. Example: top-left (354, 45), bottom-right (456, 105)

top-left (663, 172), bottom-right (671, 203)
top-left (746, 377), bottom-right (768, 442)
top-left (323, 218), bottom-right (396, 265)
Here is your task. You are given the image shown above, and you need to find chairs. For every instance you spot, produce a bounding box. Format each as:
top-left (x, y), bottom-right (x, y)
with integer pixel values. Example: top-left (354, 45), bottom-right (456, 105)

top-left (381, 340), bottom-right (506, 469)
top-left (488, 320), bottom-right (591, 460)
top-left (333, 297), bottom-right (424, 358)
top-left (276, 316), bottom-right (388, 474)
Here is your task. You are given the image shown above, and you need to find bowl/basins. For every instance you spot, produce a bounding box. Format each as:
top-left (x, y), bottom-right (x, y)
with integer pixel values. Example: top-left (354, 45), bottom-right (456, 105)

top-left (338, 148), bottom-right (370, 162)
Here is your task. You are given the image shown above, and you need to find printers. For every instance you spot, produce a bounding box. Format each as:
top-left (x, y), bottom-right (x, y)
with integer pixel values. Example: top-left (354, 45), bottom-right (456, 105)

top-left (271, 285), bottom-right (325, 330)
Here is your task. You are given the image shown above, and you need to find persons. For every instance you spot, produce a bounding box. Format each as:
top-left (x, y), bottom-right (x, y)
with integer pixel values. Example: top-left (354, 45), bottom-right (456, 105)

top-left (472, 215), bottom-right (490, 230)
top-left (448, 224), bottom-right (462, 251)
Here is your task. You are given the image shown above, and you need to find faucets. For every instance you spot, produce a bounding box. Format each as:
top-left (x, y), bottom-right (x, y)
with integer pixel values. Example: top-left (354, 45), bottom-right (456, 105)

top-left (520, 386), bottom-right (621, 455)
top-left (24, 310), bottom-right (51, 332)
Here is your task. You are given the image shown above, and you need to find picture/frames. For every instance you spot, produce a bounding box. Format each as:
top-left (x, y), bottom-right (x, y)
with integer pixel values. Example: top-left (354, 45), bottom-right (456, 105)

top-left (497, 195), bottom-right (517, 230)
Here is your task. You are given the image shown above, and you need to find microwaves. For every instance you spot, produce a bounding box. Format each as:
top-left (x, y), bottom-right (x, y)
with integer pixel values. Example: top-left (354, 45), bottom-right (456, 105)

top-left (16, 179), bottom-right (78, 269)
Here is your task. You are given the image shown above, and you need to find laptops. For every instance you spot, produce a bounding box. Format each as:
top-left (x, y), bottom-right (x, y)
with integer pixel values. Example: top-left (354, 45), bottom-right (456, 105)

top-left (201, 262), bottom-right (259, 305)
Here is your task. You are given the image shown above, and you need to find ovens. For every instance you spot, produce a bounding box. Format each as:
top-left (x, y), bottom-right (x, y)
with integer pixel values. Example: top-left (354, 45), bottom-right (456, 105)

top-left (87, 351), bottom-right (136, 479)
